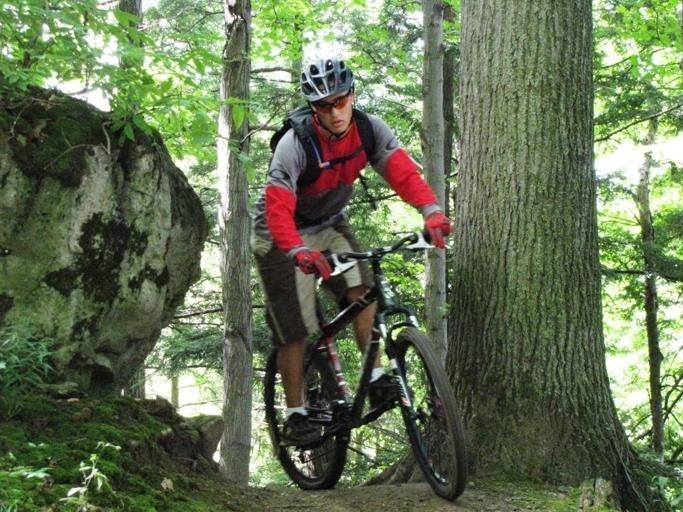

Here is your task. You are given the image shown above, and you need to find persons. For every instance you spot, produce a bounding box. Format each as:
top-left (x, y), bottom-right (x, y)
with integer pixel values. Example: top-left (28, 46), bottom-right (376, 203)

top-left (249, 58), bottom-right (451, 445)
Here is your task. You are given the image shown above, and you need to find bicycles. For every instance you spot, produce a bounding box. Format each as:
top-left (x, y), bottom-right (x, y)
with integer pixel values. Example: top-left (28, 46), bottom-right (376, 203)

top-left (262, 230), bottom-right (467, 500)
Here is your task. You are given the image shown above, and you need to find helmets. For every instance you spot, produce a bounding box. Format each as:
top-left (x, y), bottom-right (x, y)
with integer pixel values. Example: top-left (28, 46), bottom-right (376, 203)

top-left (299, 57), bottom-right (357, 102)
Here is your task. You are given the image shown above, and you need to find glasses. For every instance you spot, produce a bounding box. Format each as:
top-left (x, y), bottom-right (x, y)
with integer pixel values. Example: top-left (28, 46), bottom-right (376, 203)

top-left (308, 91), bottom-right (352, 112)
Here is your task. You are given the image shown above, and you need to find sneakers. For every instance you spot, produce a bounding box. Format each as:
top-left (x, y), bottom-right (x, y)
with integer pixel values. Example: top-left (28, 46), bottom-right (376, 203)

top-left (281, 412), bottom-right (325, 449)
top-left (369, 375), bottom-right (416, 408)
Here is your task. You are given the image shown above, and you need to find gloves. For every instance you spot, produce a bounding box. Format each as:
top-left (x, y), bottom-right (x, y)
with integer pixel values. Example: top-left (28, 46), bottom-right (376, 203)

top-left (422, 210), bottom-right (454, 248)
top-left (288, 248), bottom-right (334, 282)
